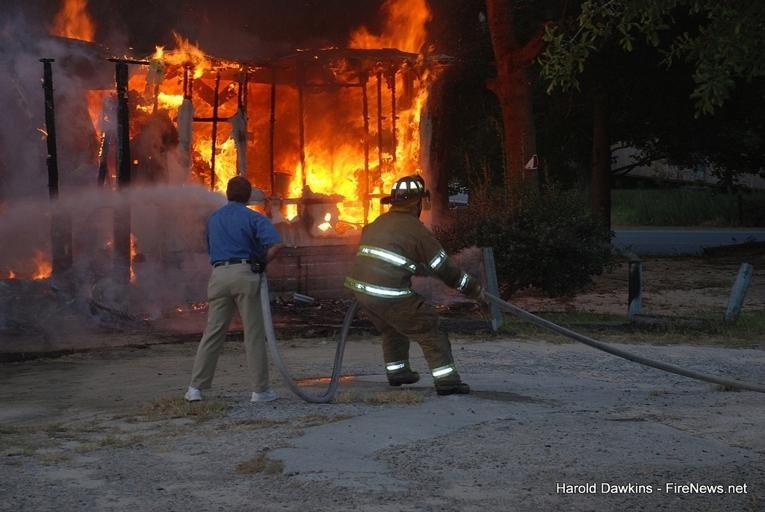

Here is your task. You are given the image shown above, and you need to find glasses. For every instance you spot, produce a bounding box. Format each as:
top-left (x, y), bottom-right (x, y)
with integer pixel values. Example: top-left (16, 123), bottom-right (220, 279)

top-left (214, 258), bottom-right (254, 267)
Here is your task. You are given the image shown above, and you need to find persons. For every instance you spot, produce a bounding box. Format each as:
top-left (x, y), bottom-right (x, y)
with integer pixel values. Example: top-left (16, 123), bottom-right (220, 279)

top-left (181, 172), bottom-right (288, 405)
top-left (340, 172), bottom-right (491, 398)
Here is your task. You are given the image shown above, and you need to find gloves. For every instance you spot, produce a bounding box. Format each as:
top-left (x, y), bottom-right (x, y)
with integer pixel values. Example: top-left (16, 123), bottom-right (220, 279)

top-left (474, 284), bottom-right (491, 306)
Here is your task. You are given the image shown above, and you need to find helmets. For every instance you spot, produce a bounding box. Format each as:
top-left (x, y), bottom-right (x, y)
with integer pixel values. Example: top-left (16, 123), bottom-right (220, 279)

top-left (380, 175), bottom-right (424, 204)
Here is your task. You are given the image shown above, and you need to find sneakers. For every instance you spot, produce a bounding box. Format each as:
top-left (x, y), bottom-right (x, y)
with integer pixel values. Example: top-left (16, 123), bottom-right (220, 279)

top-left (389, 371), bottom-right (420, 387)
top-left (184, 386), bottom-right (202, 402)
top-left (437, 382), bottom-right (469, 396)
top-left (250, 391), bottom-right (277, 402)
